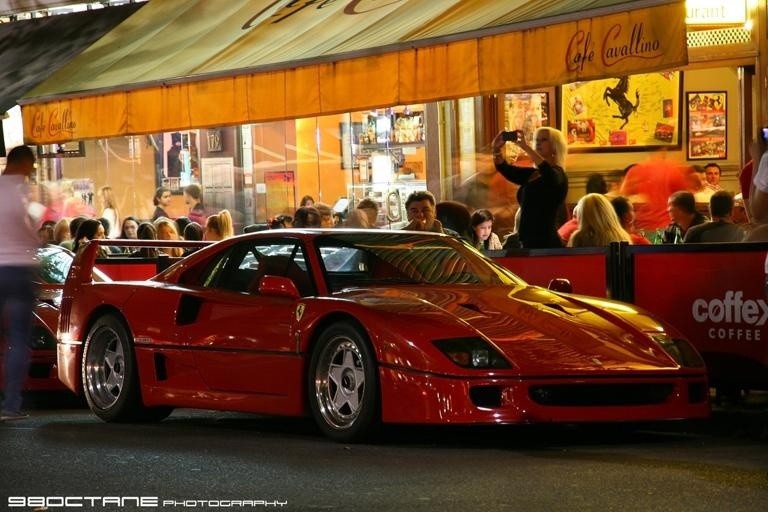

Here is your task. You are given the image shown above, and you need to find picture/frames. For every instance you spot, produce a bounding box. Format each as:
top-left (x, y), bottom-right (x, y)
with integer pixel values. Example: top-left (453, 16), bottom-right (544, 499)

top-left (555, 70), bottom-right (684, 155)
top-left (339, 122), bottom-right (363, 170)
top-left (686, 91), bottom-right (728, 161)
top-left (504, 91), bottom-right (550, 161)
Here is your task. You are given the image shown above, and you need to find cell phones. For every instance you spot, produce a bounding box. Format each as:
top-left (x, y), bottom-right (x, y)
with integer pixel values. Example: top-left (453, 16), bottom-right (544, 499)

top-left (503, 132), bottom-right (517, 141)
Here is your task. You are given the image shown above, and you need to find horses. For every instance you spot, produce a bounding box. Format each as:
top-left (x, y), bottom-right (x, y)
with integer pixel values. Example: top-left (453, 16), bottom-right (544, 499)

top-left (603, 76), bottom-right (640, 129)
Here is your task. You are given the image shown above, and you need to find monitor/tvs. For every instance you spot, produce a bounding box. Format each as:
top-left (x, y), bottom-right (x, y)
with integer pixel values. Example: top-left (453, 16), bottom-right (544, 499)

top-left (332, 197), bottom-right (353, 214)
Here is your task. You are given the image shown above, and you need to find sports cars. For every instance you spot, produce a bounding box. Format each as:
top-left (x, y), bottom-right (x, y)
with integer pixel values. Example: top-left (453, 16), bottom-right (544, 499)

top-left (57, 224), bottom-right (709, 441)
top-left (1, 240), bottom-right (116, 410)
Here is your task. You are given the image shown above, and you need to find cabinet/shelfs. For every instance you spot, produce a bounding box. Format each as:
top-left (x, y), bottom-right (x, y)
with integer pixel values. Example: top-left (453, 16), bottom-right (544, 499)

top-left (352, 142), bottom-right (427, 197)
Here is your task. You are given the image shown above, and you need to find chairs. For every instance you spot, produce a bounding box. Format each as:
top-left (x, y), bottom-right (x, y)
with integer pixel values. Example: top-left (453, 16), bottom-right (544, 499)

top-left (367, 253), bottom-right (399, 282)
top-left (249, 255), bottom-right (310, 297)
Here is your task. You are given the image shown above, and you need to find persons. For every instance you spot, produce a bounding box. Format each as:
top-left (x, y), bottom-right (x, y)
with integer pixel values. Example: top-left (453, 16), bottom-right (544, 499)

top-left (39, 126), bottom-right (768, 259)
top-left (0, 145), bottom-right (53, 421)
